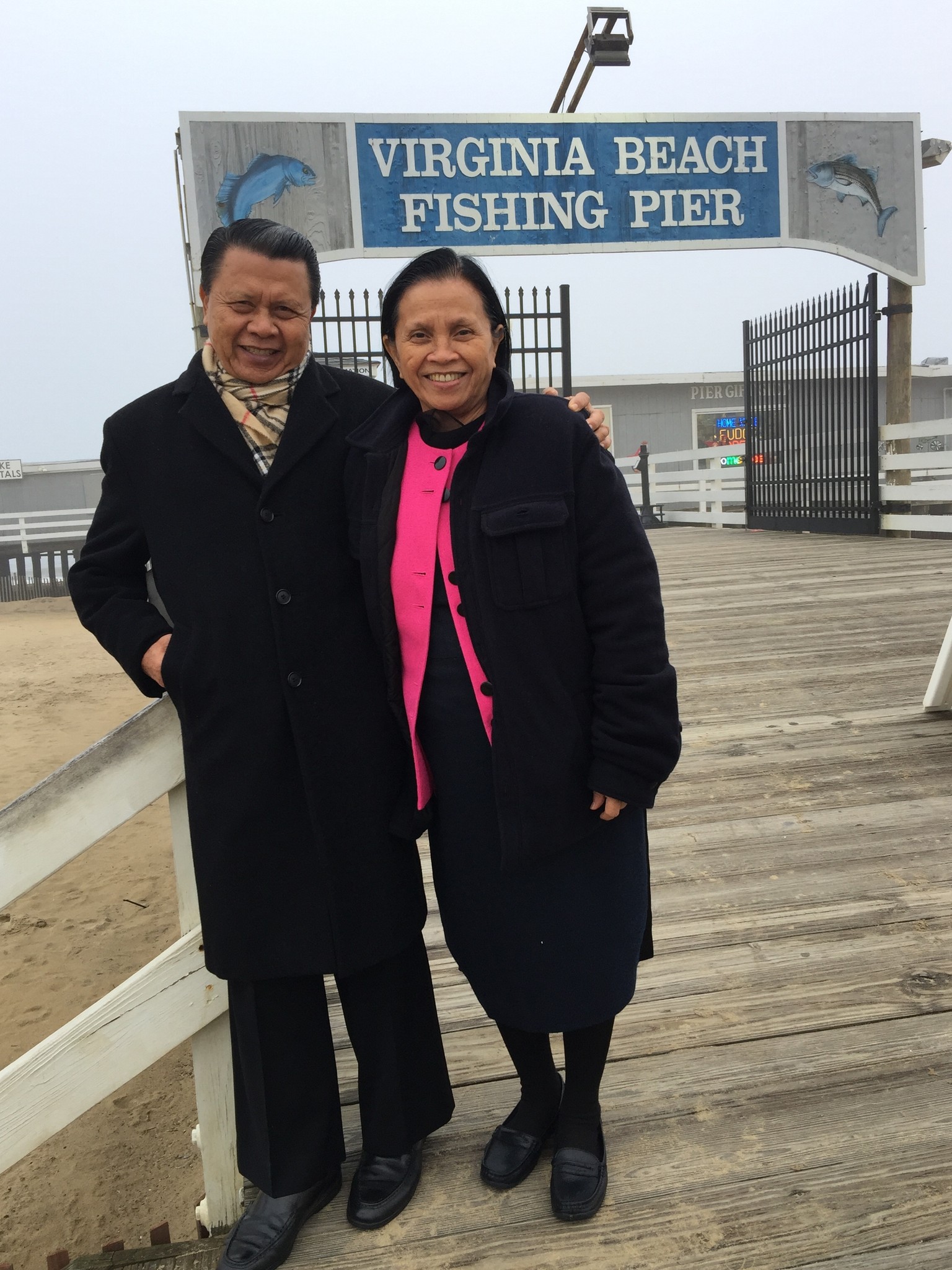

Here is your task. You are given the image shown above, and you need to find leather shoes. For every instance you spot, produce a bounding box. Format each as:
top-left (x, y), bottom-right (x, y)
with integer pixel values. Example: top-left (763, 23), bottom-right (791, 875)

top-left (551, 1097), bottom-right (610, 1221)
top-left (216, 1167), bottom-right (342, 1270)
top-left (345, 1137), bottom-right (425, 1230)
top-left (480, 1071), bottom-right (565, 1189)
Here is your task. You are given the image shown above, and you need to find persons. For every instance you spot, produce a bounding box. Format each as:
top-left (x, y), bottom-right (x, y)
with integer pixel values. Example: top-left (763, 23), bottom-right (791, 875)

top-left (65, 218), bottom-right (610, 1264)
top-left (347, 248), bottom-right (687, 1227)
